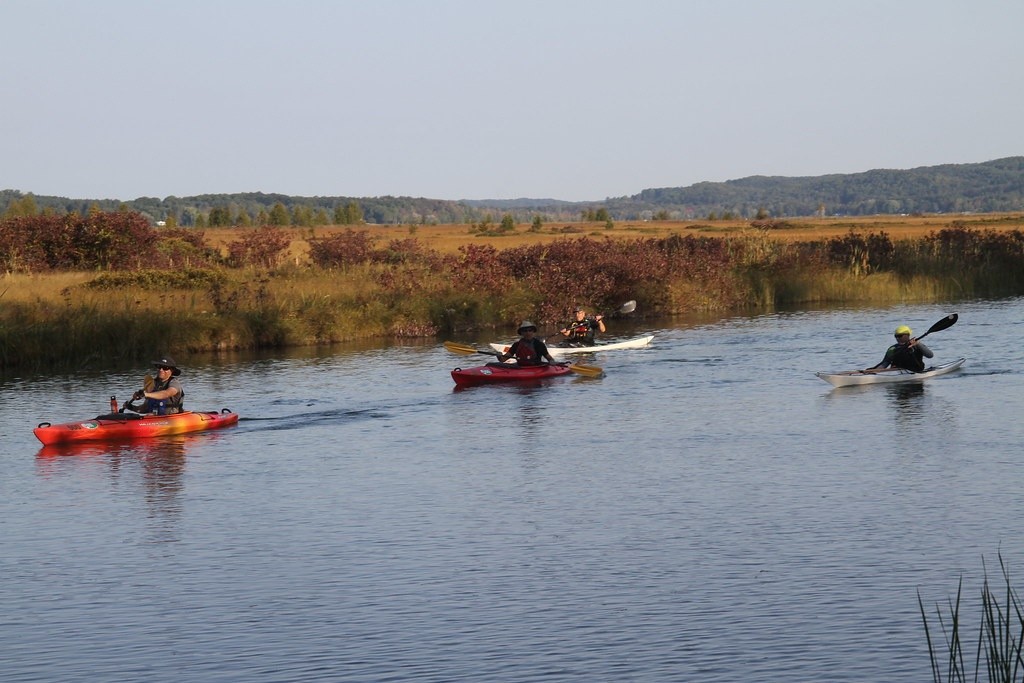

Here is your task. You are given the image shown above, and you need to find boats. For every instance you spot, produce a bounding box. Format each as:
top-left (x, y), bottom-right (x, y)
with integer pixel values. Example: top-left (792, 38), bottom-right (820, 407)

top-left (814, 357), bottom-right (968, 388)
top-left (450, 359), bottom-right (581, 385)
top-left (32, 396), bottom-right (239, 445)
top-left (489, 333), bottom-right (658, 362)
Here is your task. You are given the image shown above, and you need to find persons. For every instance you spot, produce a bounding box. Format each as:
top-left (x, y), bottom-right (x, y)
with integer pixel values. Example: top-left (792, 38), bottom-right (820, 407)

top-left (866, 326), bottom-right (934, 373)
top-left (123, 358), bottom-right (184, 415)
top-left (496, 320), bottom-right (555, 367)
top-left (559, 308), bottom-right (605, 347)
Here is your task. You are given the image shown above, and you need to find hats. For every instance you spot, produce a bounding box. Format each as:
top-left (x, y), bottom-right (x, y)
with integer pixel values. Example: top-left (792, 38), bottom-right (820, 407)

top-left (152, 356), bottom-right (181, 376)
top-left (573, 306), bottom-right (585, 313)
top-left (517, 321), bottom-right (537, 335)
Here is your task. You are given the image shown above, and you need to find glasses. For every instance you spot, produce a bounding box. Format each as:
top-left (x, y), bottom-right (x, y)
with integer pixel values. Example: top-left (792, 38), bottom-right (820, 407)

top-left (156, 364), bottom-right (170, 370)
top-left (521, 328), bottom-right (533, 332)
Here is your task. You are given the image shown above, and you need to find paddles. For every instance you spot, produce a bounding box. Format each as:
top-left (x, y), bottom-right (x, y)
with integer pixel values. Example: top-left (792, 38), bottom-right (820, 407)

top-left (119, 375), bottom-right (155, 413)
top-left (869, 313), bottom-right (959, 372)
top-left (444, 341), bottom-right (602, 379)
top-left (542, 299), bottom-right (636, 340)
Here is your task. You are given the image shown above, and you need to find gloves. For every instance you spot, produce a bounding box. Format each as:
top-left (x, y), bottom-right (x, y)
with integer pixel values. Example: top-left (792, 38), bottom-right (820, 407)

top-left (549, 359), bottom-right (555, 365)
top-left (123, 401), bottom-right (132, 410)
top-left (133, 389), bottom-right (144, 400)
